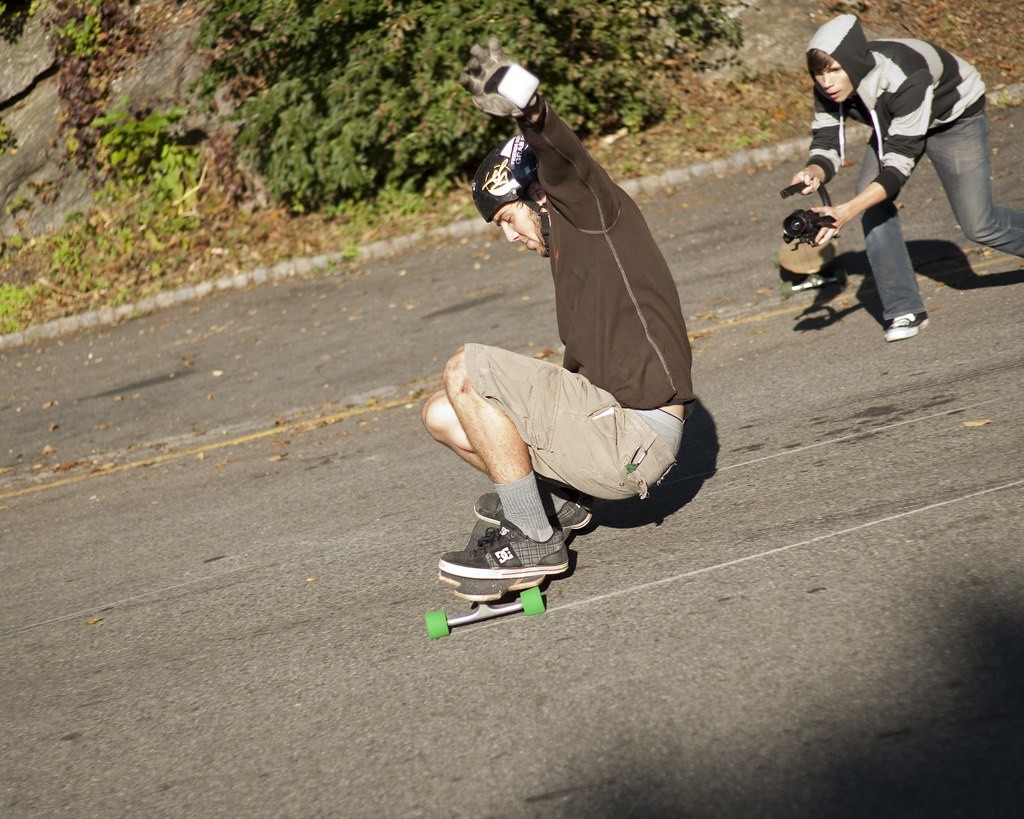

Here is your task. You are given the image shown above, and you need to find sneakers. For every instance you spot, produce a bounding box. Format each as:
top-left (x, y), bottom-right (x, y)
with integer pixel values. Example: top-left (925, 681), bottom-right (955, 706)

top-left (438, 517), bottom-right (570, 579)
top-left (472, 487), bottom-right (593, 532)
top-left (885, 312), bottom-right (931, 344)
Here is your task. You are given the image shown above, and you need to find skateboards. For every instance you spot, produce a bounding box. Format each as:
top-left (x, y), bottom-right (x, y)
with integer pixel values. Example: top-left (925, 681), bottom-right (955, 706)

top-left (770, 232), bottom-right (847, 296)
top-left (424, 518), bottom-right (573, 644)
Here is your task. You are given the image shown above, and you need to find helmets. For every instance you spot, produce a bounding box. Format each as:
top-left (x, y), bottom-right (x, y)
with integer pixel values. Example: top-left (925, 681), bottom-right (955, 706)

top-left (471, 139), bottom-right (543, 223)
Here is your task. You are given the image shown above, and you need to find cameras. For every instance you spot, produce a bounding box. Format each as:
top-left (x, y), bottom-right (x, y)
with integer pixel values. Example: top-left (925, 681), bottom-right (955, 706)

top-left (782, 209), bottom-right (820, 247)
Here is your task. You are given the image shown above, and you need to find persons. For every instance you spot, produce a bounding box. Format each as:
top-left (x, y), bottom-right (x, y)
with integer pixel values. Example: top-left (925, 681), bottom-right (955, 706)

top-left (793, 15), bottom-right (1023, 342)
top-left (420, 33), bottom-right (696, 581)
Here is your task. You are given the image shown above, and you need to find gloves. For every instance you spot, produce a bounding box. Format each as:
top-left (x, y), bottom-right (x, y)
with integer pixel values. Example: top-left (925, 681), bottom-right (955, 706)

top-left (458, 37), bottom-right (545, 117)
